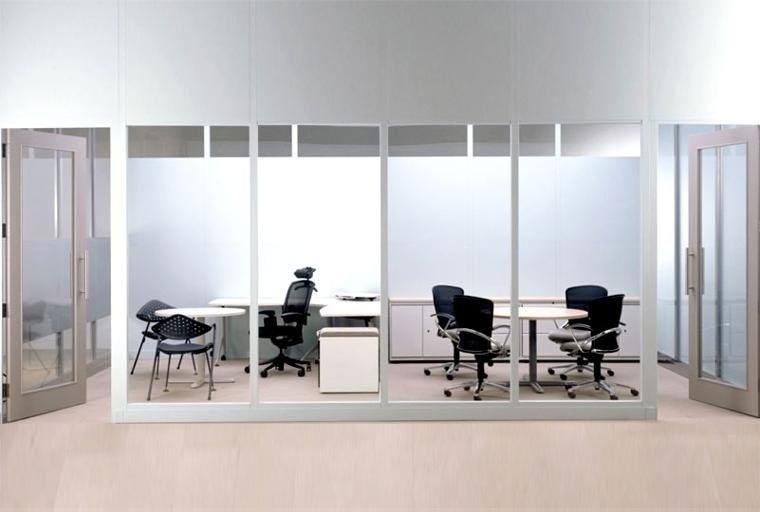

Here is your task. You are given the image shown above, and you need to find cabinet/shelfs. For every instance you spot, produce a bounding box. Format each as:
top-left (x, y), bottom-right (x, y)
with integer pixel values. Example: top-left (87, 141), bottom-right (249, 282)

top-left (388, 302), bottom-right (640, 362)
top-left (318, 326), bottom-right (379, 394)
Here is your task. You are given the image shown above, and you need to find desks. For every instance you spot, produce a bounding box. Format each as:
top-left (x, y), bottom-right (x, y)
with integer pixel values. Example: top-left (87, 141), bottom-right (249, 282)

top-left (206, 297), bottom-right (381, 367)
top-left (153, 307), bottom-right (246, 387)
top-left (489, 306), bottom-right (589, 393)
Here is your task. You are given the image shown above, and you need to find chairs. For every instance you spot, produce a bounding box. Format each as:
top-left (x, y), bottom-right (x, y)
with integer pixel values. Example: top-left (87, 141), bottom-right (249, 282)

top-left (547, 285), bottom-right (639, 400)
top-left (244, 267), bottom-right (315, 376)
top-left (146, 314), bottom-right (216, 401)
top-left (423, 285), bottom-right (510, 401)
top-left (129, 299), bottom-right (198, 379)
